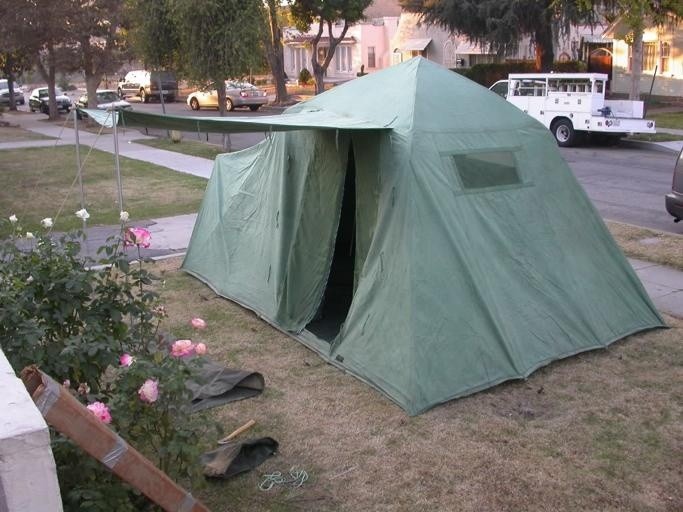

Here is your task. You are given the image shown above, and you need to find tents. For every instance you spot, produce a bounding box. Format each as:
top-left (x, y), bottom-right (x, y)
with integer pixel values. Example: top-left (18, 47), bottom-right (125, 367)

top-left (79, 53), bottom-right (673, 418)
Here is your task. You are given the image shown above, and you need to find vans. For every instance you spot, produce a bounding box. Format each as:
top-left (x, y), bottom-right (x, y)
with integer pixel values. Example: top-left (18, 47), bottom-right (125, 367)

top-left (0, 77), bottom-right (24, 107)
top-left (116, 68), bottom-right (179, 104)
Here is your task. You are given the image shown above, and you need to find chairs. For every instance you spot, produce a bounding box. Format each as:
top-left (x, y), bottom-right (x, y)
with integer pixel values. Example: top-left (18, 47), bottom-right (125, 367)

top-left (558, 83), bottom-right (599, 93)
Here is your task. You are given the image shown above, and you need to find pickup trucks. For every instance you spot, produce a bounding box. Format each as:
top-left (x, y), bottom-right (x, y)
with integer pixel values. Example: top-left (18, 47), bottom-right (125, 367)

top-left (487, 70), bottom-right (657, 148)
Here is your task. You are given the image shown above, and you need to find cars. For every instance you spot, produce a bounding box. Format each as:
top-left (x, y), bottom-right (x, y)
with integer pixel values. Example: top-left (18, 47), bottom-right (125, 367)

top-left (27, 87), bottom-right (72, 115)
top-left (185, 79), bottom-right (268, 112)
top-left (74, 89), bottom-right (132, 120)
